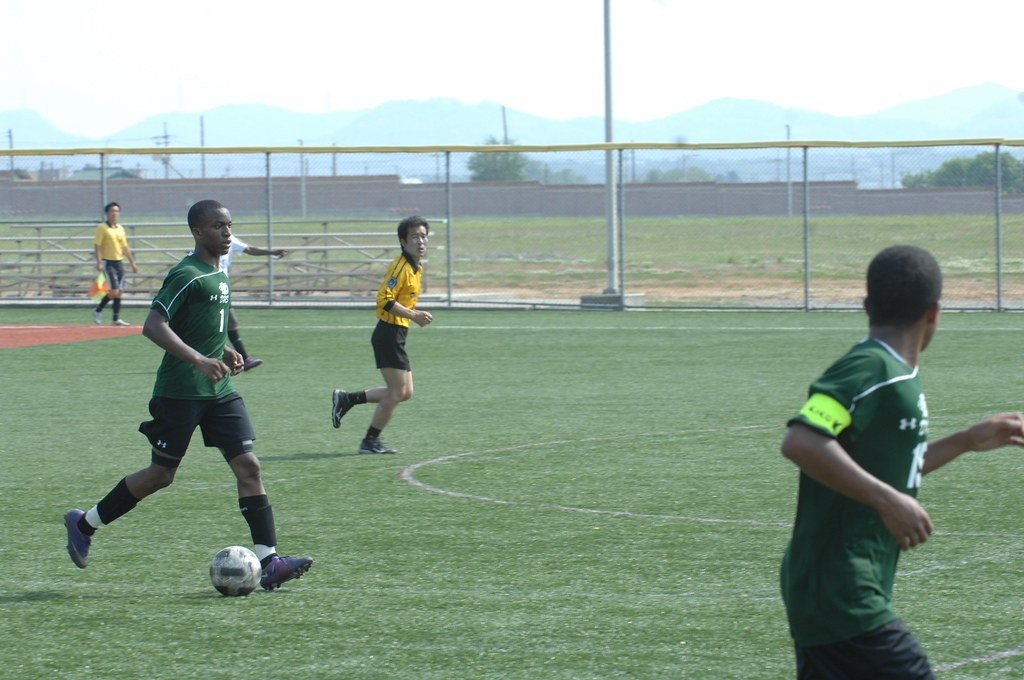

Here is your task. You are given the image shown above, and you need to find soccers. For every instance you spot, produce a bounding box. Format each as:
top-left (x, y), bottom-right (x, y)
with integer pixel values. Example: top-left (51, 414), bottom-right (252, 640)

top-left (209, 545), bottom-right (262, 597)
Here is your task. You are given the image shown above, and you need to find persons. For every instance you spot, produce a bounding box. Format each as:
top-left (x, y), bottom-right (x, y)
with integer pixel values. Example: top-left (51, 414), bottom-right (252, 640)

top-left (91, 202), bottom-right (138, 325)
top-left (331, 216), bottom-right (434, 453)
top-left (63, 199), bottom-right (313, 591)
top-left (779, 243), bottom-right (1024, 680)
top-left (187, 232), bottom-right (288, 370)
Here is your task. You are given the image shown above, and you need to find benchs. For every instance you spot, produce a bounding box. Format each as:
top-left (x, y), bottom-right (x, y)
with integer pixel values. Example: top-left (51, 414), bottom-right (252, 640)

top-left (0, 218), bottom-right (446, 301)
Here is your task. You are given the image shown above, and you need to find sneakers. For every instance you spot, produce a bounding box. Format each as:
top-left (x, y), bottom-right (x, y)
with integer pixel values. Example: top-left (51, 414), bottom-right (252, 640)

top-left (64, 509), bottom-right (91, 569)
top-left (358, 438), bottom-right (398, 455)
top-left (91, 308), bottom-right (101, 324)
top-left (259, 555), bottom-right (314, 591)
top-left (244, 357), bottom-right (263, 372)
top-left (331, 388), bottom-right (355, 429)
top-left (112, 317), bottom-right (130, 326)
